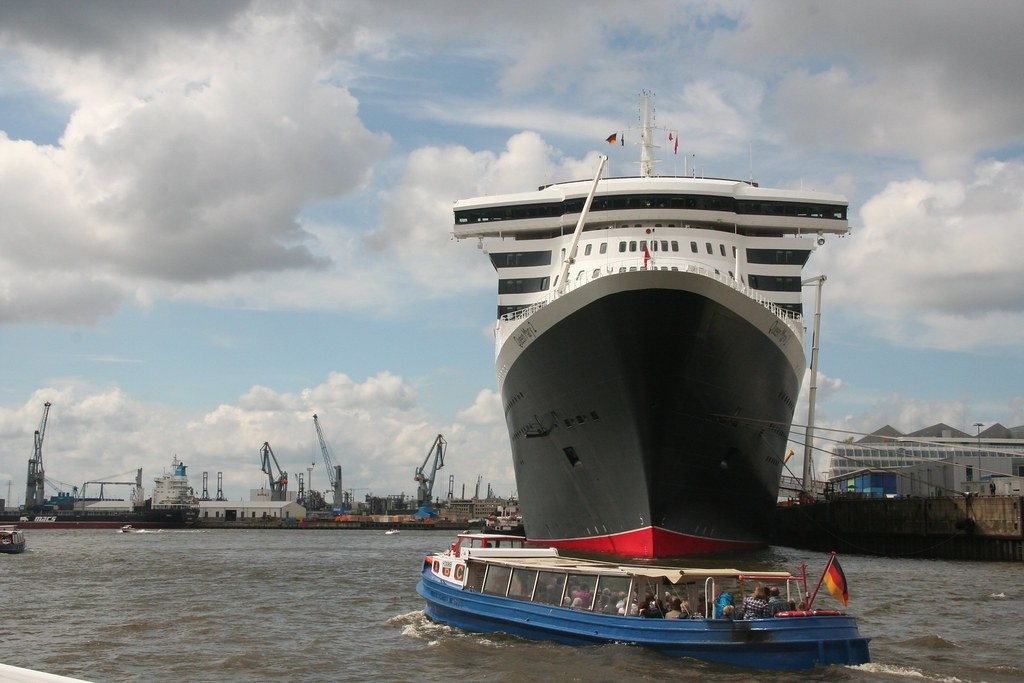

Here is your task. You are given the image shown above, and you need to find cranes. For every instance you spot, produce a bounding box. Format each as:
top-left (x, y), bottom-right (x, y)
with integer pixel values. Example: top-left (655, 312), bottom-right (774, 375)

top-left (23, 400), bottom-right (51, 507)
top-left (410, 434), bottom-right (447, 520)
top-left (259, 441), bottom-right (288, 501)
top-left (310, 413), bottom-right (345, 511)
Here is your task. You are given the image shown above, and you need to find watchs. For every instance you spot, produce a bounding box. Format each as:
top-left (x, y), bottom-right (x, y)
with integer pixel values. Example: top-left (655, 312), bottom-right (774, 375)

top-left (799, 602), bottom-right (808, 611)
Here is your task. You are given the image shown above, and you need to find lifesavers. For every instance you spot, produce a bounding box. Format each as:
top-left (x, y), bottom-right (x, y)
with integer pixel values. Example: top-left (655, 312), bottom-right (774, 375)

top-left (814, 610), bottom-right (843, 615)
top-left (776, 610), bottom-right (812, 616)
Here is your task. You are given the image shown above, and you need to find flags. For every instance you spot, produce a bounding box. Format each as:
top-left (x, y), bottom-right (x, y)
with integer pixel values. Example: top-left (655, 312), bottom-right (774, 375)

top-left (674, 134), bottom-right (679, 155)
top-left (643, 247), bottom-right (651, 270)
top-left (823, 556), bottom-right (849, 607)
top-left (606, 133), bottom-right (616, 145)
top-left (668, 133), bottom-right (673, 141)
top-left (621, 133), bottom-right (625, 147)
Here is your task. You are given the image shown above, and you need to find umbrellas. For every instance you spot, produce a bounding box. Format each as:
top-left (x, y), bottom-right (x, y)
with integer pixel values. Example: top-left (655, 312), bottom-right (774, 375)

top-left (846, 485), bottom-right (858, 489)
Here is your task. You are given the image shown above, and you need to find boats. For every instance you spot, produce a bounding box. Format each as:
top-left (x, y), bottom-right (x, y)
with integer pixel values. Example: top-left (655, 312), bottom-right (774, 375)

top-left (0, 525), bottom-right (25, 553)
top-left (410, 546), bottom-right (872, 671)
top-left (122, 524), bottom-right (133, 532)
top-left (480, 489), bottom-right (525, 537)
top-left (448, 529), bottom-right (530, 562)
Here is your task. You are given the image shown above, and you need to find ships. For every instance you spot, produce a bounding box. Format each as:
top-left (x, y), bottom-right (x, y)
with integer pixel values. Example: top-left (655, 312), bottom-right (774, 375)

top-left (449, 83), bottom-right (853, 568)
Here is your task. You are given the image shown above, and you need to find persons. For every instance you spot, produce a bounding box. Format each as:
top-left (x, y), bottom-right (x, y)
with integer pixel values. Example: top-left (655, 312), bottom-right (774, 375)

top-left (742, 586), bottom-right (770, 619)
top-left (719, 605), bottom-right (735, 619)
top-left (989, 480), bottom-right (996, 496)
top-left (484, 567), bottom-right (702, 620)
top-left (713, 585), bottom-right (734, 618)
top-left (766, 586), bottom-right (790, 616)
top-left (784, 599), bottom-right (797, 612)
